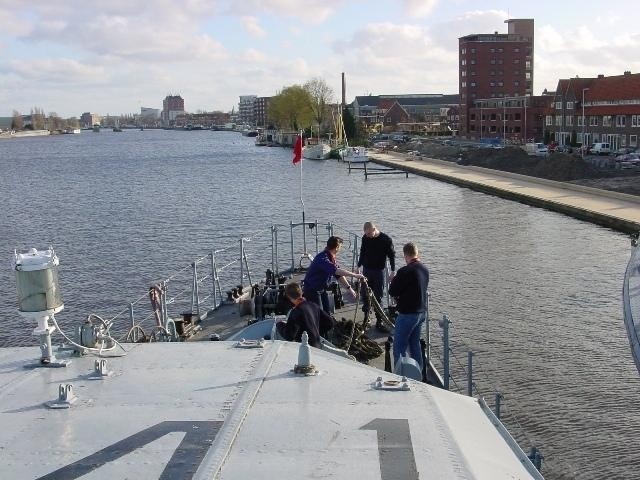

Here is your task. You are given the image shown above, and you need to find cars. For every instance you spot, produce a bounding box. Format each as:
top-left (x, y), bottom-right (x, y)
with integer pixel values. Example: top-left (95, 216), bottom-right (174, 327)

top-left (524, 139), bottom-right (640, 169)
top-left (370, 132), bottom-right (503, 154)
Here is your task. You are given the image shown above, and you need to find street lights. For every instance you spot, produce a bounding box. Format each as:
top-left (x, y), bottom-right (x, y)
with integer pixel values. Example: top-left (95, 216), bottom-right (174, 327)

top-left (525, 94), bottom-right (530, 143)
top-left (503, 93), bottom-right (509, 145)
top-left (582, 88), bottom-right (590, 159)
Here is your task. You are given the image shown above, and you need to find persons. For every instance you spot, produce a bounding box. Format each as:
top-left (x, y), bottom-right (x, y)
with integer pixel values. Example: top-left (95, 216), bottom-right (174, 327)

top-left (354, 219), bottom-right (396, 334)
top-left (304, 234), bottom-right (363, 315)
top-left (387, 241), bottom-right (429, 372)
top-left (273, 281), bottom-right (333, 348)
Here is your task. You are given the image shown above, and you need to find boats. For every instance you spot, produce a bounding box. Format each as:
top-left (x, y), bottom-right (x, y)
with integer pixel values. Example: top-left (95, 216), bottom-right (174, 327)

top-left (0, 212), bottom-right (556, 480)
top-left (210, 101), bottom-right (372, 163)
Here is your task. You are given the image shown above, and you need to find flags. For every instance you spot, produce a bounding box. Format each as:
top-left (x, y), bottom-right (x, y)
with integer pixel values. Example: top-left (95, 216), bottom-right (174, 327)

top-left (291, 133), bottom-right (302, 165)
